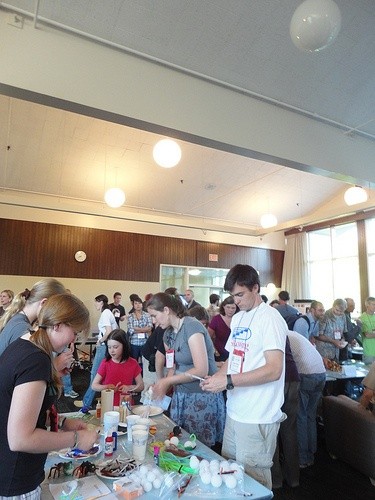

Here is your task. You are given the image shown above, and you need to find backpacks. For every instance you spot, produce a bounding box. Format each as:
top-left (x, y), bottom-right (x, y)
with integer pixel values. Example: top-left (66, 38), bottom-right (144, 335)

top-left (288, 315), bottom-right (311, 336)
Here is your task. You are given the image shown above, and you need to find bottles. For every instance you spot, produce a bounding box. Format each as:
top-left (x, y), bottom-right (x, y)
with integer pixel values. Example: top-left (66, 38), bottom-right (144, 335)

top-left (96, 399), bottom-right (102, 419)
top-left (119, 402), bottom-right (128, 425)
top-left (112, 428), bottom-right (118, 450)
top-left (104, 432), bottom-right (114, 457)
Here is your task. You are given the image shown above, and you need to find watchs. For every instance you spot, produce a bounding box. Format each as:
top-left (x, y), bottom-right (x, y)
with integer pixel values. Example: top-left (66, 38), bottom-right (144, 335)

top-left (227, 374), bottom-right (234, 390)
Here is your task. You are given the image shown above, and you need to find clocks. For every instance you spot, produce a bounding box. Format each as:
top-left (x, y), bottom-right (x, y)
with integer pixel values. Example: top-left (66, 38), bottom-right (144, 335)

top-left (74, 250), bottom-right (87, 263)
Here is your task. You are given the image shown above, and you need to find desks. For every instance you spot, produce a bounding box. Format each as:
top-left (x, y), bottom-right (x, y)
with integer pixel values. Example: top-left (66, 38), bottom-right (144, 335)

top-left (316, 358), bottom-right (371, 381)
top-left (38, 404), bottom-right (274, 500)
top-left (85, 337), bottom-right (98, 364)
top-left (72, 341), bottom-right (82, 364)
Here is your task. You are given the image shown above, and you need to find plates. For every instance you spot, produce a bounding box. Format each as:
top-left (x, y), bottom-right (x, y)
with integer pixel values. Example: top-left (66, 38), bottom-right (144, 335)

top-left (131, 405), bottom-right (164, 417)
top-left (95, 458), bottom-right (144, 480)
top-left (95, 422), bottom-right (129, 437)
top-left (353, 347), bottom-right (364, 351)
top-left (58, 442), bottom-right (102, 459)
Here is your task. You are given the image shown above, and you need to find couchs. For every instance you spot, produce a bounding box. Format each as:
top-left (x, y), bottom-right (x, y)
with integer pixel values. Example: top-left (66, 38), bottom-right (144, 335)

top-left (322, 393), bottom-right (375, 482)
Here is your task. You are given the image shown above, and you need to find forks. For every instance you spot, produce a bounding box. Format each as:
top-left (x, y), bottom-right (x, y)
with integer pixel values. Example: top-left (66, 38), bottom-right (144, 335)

top-left (98, 426), bottom-right (101, 434)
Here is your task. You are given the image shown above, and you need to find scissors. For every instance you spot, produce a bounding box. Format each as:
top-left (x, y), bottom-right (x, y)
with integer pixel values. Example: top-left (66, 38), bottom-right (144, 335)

top-left (184, 372), bottom-right (206, 382)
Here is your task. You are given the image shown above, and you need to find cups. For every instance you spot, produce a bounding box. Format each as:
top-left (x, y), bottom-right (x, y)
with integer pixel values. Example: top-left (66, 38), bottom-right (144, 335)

top-left (131, 429), bottom-right (149, 461)
top-left (126, 415), bottom-right (141, 441)
top-left (103, 411), bottom-right (119, 445)
top-left (131, 425), bottom-right (148, 433)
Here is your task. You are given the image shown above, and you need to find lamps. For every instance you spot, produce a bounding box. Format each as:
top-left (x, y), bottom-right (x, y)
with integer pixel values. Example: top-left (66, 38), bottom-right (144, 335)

top-left (152, 138), bottom-right (182, 169)
top-left (344, 185), bottom-right (368, 206)
top-left (289, 0), bottom-right (343, 55)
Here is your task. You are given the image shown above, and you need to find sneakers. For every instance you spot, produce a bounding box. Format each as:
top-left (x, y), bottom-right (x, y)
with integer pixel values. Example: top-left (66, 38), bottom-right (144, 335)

top-left (64, 389), bottom-right (79, 399)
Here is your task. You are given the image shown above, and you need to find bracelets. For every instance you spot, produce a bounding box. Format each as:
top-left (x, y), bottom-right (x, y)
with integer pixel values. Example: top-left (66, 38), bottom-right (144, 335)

top-left (57, 415), bottom-right (66, 429)
top-left (72, 430), bottom-right (80, 449)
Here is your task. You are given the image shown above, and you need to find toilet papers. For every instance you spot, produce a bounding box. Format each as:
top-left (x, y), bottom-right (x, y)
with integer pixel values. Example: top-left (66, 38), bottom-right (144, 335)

top-left (100, 389), bottom-right (115, 426)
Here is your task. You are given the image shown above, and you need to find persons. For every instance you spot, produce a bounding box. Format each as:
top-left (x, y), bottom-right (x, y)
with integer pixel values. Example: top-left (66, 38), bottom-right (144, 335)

top-left (199, 264), bottom-right (288, 492)
top-left (75, 294), bottom-right (121, 414)
top-left (279, 329), bottom-right (328, 479)
top-left (293, 301), bottom-right (324, 353)
top-left (267, 332), bottom-right (299, 490)
top-left (0, 290), bottom-right (14, 317)
top-left (128, 301), bottom-right (153, 366)
top-left (146, 291), bottom-right (226, 450)
top-left (356, 296), bottom-right (375, 365)
top-left (106, 282), bottom-right (305, 329)
top-left (90, 329), bottom-right (144, 407)
top-left (360, 358), bottom-right (375, 487)
top-left (1, 279), bottom-right (74, 410)
top-left (205, 296), bottom-right (241, 362)
top-left (313, 299), bottom-right (348, 363)
top-left (339, 298), bottom-right (361, 360)
top-left (0, 292), bottom-right (100, 499)
